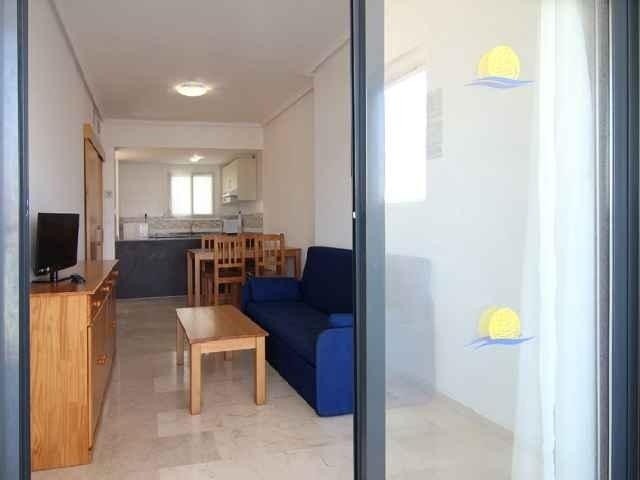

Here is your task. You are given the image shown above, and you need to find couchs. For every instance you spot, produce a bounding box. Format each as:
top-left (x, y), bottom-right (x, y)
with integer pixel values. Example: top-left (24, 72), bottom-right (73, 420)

top-left (242, 245), bottom-right (438, 418)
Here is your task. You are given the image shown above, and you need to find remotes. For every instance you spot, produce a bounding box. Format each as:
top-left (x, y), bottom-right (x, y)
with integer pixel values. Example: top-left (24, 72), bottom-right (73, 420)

top-left (70, 273), bottom-right (86, 283)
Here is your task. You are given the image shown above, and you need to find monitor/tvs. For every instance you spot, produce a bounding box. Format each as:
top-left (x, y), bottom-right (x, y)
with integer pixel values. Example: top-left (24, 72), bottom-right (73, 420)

top-left (32, 212), bottom-right (80, 283)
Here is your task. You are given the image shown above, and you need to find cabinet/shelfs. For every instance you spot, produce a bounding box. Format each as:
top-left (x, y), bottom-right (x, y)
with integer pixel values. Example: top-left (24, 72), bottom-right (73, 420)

top-left (28, 258), bottom-right (122, 473)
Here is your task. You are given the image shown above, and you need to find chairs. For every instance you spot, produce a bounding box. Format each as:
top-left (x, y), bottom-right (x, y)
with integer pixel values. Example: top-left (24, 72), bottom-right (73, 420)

top-left (200, 232), bottom-right (285, 306)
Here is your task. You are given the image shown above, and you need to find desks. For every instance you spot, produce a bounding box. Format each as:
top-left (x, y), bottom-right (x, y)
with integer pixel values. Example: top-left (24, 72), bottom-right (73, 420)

top-left (183, 246), bottom-right (303, 308)
